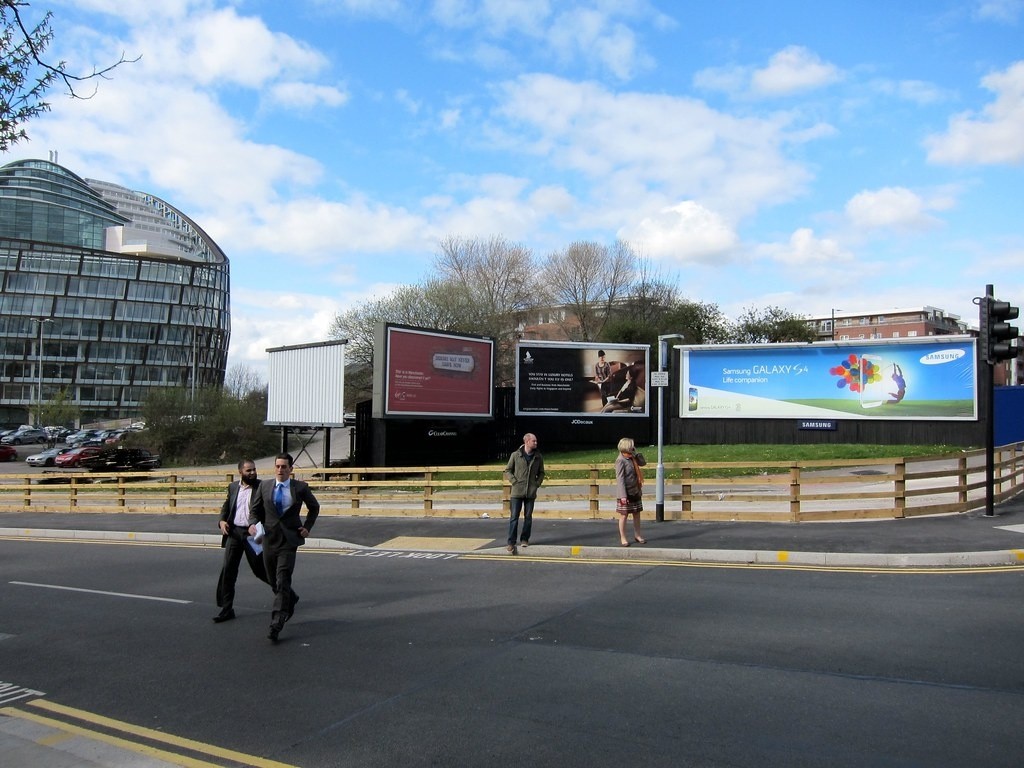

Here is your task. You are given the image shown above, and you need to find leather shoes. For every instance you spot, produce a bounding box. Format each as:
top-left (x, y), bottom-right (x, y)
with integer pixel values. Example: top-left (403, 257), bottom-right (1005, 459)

top-left (266, 626), bottom-right (285, 646)
top-left (284, 590), bottom-right (299, 622)
top-left (214, 609), bottom-right (235, 623)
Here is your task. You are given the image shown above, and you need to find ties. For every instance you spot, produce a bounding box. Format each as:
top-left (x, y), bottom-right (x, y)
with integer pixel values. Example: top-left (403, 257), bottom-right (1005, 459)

top-left (276, 484), bottom-right (283, 513)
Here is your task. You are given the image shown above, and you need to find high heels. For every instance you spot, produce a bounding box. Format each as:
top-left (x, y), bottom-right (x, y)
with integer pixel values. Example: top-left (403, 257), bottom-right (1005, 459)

top-left (622, 542), bottom-right (629, 547)
top-left (635, 538), bottom-right (645, 544)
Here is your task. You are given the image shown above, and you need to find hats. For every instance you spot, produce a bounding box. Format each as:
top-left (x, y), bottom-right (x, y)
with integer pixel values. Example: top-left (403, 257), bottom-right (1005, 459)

top-left (599, 350), bottom-right (605, 357)
top-left (629, 369), bottom-right (639, 379)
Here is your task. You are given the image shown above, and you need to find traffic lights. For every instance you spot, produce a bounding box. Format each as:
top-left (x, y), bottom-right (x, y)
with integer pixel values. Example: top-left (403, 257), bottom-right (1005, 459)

top-left (997, 307), bottom-right (1020, 364)
top-left (980, 298), bottom-right (1011, 361)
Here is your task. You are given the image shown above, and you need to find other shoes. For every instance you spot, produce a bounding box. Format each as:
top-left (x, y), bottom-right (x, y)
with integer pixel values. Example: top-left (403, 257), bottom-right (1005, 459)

top-left (521, 540), bottom-right (528, 547)
top-left (506, 545), bottom-right (515, 553)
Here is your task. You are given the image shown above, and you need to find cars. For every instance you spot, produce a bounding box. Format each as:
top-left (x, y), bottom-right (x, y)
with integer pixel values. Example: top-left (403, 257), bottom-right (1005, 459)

top-left (44, 422), bottom-right (149, 449)
top-left (55, 447), bottom-right (101, 468)
top-left (0, 429), bottom-right (48, 446)
top-left (0, 445), bottom-right (19, 462)
top-left (343, 413), bottom-right (356, 428)
top-left (0, 424), bottom-right (43, 440)
top-left (26, 446), bottom-right (73, 468)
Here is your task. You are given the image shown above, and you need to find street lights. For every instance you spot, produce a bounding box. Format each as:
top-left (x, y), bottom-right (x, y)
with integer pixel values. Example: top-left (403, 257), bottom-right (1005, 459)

top-left (190, 305), bottom-right (204, 422)
top-left (30, 318), bottom-right (54, 424)
top-left (656, 334), bottom-right (684, 521)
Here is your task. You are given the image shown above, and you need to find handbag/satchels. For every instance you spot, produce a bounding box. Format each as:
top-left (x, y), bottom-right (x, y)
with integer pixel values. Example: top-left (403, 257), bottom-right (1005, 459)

top-left (634, 453), bottom-right (646, 466)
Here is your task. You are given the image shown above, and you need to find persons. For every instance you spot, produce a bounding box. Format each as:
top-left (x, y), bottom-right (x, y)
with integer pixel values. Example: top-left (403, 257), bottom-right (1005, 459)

top-left (886, 363), bottom-right (906, 404)
top-left (212, 459), bottom-right (300, 623)
top-left (505, 433), bottom-right (545, 552)
top-left (601, 368), bottom-right (638, 413)
top-left (248, 453), bottom-right (320, 639)
top-left (595, 350), bottom-right (612, 407)
top-left (615, 438), bottom-right (645, 546)
